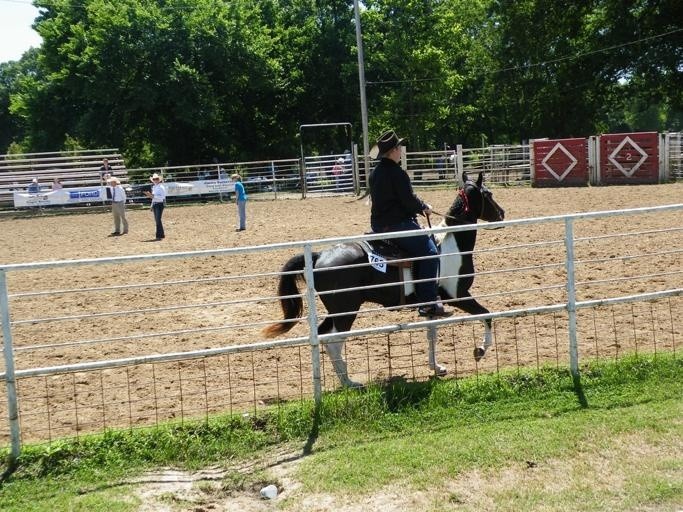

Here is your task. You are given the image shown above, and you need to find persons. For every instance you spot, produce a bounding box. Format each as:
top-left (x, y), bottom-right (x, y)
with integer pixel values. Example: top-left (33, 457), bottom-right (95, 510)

top-left (344, 149), bottom-right (352, 163)
top-left (332, 163), bottom-right (343, 188)
top-left (369, 130), bottom-right (455, 317)
top-left (52, 177), bottom-right (62, 191)
top-left (150, 173), bottom-right (166, 241)
top-left (100, 159), bottom-right (112, 186)
top-left (436, 155), bottom-right (444, 179)
top-left (28, 179), bottom-right (41, 193)
top-left (197, 167), bottom-right (210, 197)
top-left (220, 168), bottom-right (229, 179)
top-left (231, 173), bottom-right (247, 232)
top-left (107, 177), bottom-right (128, 235)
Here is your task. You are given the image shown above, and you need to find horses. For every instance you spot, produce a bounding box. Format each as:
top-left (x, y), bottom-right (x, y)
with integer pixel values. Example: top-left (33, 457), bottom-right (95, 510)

top-left (264, 171), bottom-right (507, 391)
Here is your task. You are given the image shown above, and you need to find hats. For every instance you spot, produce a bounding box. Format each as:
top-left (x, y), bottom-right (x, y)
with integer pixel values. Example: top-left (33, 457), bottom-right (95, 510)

top-left (369, 130), bottom-right (408, 159)
top-left (150, 173), bottom-right (162, 182)
top-left (107, 177), bottom-right (120, 184)
top-left (230, 174), bottom-right (240, 179)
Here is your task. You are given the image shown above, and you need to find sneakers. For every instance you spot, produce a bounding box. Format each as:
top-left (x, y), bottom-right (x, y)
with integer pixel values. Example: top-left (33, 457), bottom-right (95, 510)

top-left (418, 304), bottom-right (453, 319)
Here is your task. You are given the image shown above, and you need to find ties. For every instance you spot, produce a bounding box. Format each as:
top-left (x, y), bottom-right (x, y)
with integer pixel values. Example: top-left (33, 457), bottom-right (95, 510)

top-left (112, 187), bottom-right (115, 201)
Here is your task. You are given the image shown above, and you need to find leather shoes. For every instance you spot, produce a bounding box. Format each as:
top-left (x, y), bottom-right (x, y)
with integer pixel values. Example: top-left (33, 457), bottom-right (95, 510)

top-left (110, 232), bottom-right (128, 235)
top-left (155, 236), bottom-right (165, 241)
top-left (236, 228), bottom-right (246, 232)
